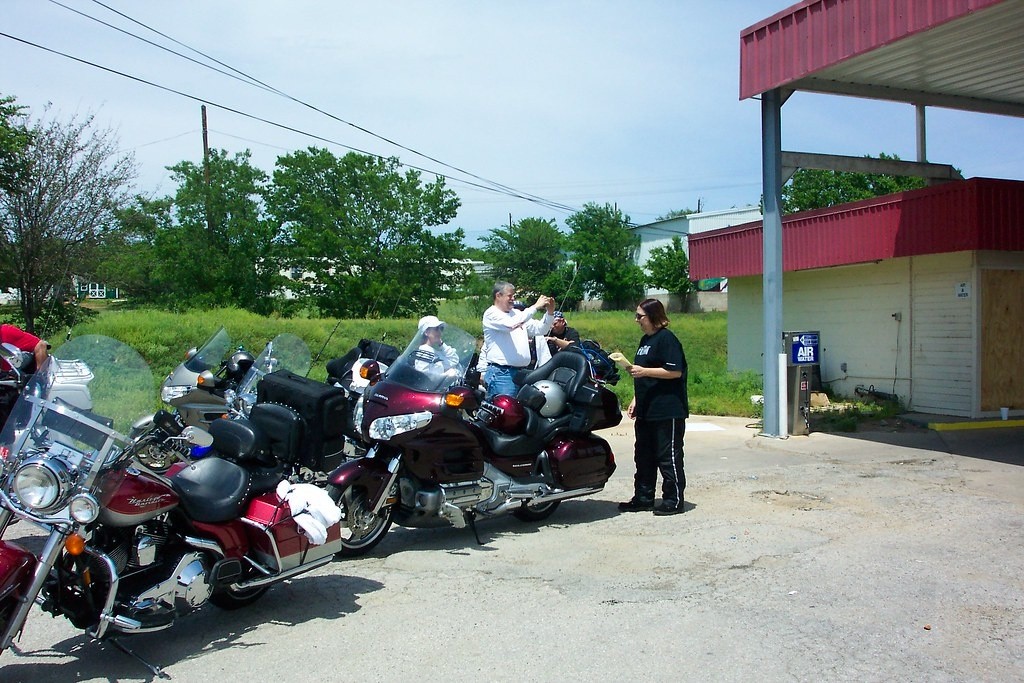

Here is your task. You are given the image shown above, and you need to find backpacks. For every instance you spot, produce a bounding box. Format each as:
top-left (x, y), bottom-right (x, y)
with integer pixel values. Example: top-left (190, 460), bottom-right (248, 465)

top-left (580, 339), bottom-right (620, 386)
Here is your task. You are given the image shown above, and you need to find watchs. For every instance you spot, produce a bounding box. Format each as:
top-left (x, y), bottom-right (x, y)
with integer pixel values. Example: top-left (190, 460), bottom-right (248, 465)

top-left (37, 371), bottom-right (47, 379)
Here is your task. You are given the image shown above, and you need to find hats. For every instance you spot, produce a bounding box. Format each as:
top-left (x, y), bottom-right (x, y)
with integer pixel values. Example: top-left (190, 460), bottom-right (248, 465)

top-left (418, 315), bottom-right (446, 335)
top-left (553, 311), bottom-right (568, 325)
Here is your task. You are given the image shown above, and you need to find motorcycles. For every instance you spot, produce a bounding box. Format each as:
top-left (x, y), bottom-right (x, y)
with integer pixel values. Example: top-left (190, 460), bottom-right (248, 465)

top-left (1, 319), bottom-right (623, 680)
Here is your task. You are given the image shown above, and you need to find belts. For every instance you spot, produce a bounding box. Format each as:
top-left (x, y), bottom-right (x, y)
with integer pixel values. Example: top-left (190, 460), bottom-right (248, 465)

top-left (488, 363), bottom-right (528, 370)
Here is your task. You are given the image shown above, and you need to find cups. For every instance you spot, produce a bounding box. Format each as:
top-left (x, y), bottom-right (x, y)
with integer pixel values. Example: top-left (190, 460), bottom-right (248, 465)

top-left (1001, 408), bottom-right (1010, 420)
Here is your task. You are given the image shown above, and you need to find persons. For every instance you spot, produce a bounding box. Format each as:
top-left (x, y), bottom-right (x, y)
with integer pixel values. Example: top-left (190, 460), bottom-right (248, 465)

top-left (0, 324), bottom-right (48, 385)
top-left (618, 299), bottom-right (688, 516)
top-left (414, 316), bottom-right (462, 390)
top-left (475, 281), bottom-right (579, 401)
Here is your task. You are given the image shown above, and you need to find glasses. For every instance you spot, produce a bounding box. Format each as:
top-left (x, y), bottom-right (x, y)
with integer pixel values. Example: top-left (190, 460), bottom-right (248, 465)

top-left (635, 313), bottom-right (654, 320)
top-left (435, 326), bottom-right (445, 332)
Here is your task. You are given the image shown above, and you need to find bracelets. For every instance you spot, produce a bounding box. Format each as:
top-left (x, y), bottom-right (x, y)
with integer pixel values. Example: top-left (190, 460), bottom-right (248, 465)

top-left (438, 342), bottom-right (445, 347)
top-left (552, 337), bottom-right (557, 343)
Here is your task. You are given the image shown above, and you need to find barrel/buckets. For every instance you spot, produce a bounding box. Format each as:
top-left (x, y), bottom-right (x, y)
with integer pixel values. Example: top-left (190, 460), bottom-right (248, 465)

top-left (785, 331), bottom-right (819, 364)
top-left (749, 393), bottom-right (764, 406)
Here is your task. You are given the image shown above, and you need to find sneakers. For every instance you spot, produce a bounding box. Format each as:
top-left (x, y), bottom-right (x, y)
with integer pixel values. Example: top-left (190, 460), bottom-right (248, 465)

top-left (617, 497), bottom-right (655, 512)
top-left (652, 503), bottom-right (686, 514)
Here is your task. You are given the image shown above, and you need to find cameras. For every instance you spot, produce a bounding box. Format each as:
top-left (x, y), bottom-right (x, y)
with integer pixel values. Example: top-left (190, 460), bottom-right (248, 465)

top-left (544, 297), bottom-right (552, 307)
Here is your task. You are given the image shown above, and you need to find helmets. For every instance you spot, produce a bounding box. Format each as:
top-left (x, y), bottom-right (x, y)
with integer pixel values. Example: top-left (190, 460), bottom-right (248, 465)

top-left (228, 353), bottom-right (255, 372)
top-left (532, 380), bottom-right (567, 418)
top-left (487, 393), bottom-right (525, 435)
